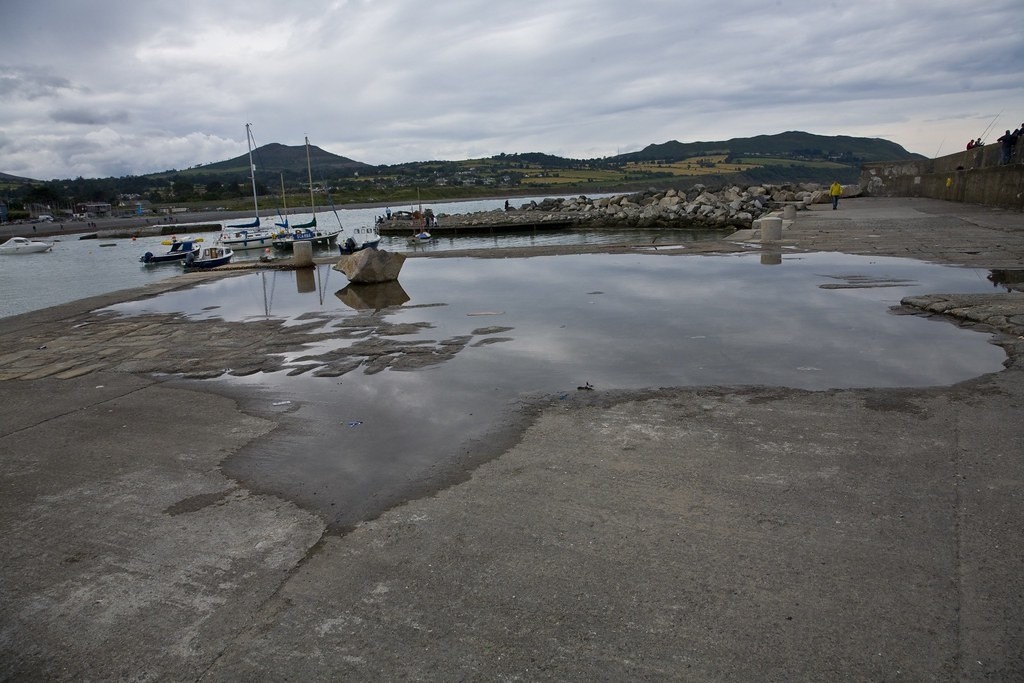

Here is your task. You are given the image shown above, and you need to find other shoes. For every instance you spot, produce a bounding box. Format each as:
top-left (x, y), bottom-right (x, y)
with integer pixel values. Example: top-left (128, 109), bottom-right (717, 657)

top-left (832, 207), bottom-right (838, 210)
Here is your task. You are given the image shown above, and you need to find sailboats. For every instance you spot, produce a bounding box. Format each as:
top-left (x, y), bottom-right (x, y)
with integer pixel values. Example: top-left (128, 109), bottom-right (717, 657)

top-left (338, 215), bottom-right (381, 256)
top-left (216, 121), bottom-right (344, 252)
top-left (406, 186), bottom-right (433, 246)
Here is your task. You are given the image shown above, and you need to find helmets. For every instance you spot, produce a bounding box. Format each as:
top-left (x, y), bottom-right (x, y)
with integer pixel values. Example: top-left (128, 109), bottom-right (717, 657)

top-left (833, 177), bottom-right (839, 181)
top-left (978, 138), bottom-right (981, 140)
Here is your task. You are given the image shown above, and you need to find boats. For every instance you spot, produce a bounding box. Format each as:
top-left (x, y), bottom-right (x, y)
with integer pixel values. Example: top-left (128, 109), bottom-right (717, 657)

top-left (0, 237), bottom-right (54, 255)
top-left (79, 223), bottom-right (222, 242)
top-left (180, 245), bottom-right (234, 268)
top-left (137, 236), bottom-right (204, 263)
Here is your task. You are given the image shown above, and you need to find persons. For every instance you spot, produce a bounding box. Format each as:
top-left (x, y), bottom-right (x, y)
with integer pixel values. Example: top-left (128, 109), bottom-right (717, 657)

top-left (967, 140), bottom-right (974, 150)
top-left (426, 214), bottom-right (438, 227)
top-left (1013, 123), bottom-right (1024, 136)
top-left (829, 179), bottom-right (843, 210)
top-left (997, 130), bottom-right (1016, 165)
top-left (975, 138), bottom-right (985, 147)
top-left (386, 207), bottom-right (391, 219)
top-left (505, 200), bottom-right (510, 210)
